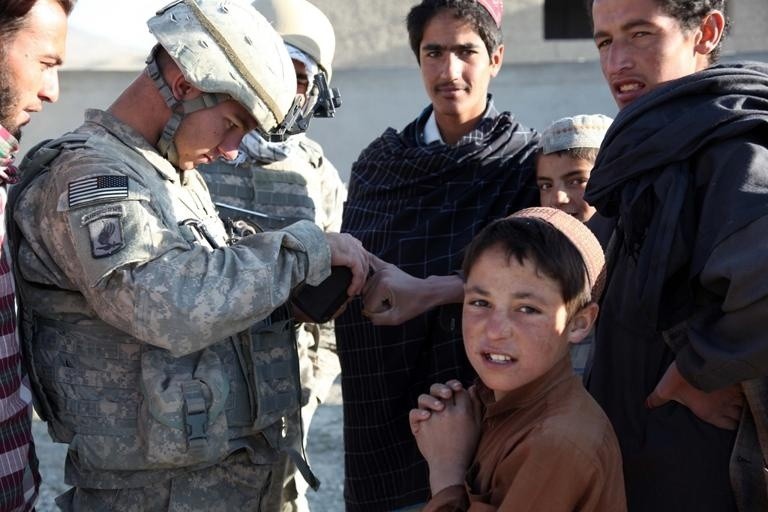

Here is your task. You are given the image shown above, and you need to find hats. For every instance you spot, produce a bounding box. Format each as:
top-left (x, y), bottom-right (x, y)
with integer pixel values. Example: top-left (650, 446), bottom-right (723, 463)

top-left (508, 206), bottom-right (608, 306)
top-left (476, 0), bottom-right (508, 29)
top-left (541, 112), bottom-right (613, 157)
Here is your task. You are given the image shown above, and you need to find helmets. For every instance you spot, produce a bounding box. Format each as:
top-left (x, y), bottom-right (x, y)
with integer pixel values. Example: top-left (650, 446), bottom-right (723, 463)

top-left (147, 0), bottom-right (342, 142)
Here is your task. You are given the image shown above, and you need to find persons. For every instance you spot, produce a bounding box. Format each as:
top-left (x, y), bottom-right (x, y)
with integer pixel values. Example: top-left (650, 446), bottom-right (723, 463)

top-left (195, 0), bottom-right (351, 511)
top-left (7, 0), bottom-right (374, 511)
top-left (358, 0), bottom-right (766, 510)
top-left (535, 111), bottom-right (619, 387)
top-left (332, 0), bottom-right (547, 511)
top-left (407, 204), bottom-right (629, 510)
top-left (0, 0), bottom-right (80, 511)
